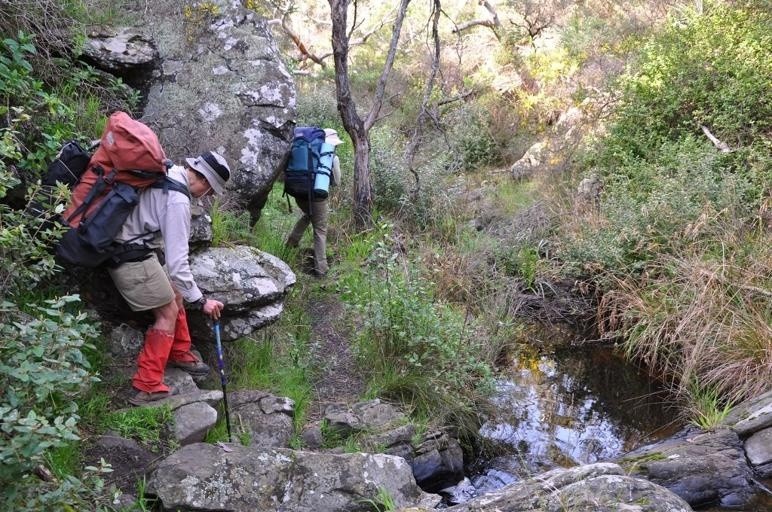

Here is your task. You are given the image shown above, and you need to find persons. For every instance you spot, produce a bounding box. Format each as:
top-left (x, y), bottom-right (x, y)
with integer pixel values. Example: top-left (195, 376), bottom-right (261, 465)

top-left (94, 151), bottom-right (231, 408)
top-left (286, 128), bottom-right (346, 279)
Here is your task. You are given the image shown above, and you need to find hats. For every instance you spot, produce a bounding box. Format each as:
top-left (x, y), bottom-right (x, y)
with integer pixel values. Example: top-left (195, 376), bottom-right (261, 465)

top-left (321, 128), bottom-right (345, 147)
top-left (185, 151), bottom-right (231, 197)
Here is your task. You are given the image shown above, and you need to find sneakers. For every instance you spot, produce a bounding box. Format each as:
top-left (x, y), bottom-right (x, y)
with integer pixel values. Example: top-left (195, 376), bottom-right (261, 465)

top-left (126, 385), bottom-right (180, 406)
top-left (166, 354), bottom-right (212, 377)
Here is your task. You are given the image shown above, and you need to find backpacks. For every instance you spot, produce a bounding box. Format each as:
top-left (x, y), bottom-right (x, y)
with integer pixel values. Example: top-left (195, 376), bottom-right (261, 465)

top-left (283, 126), bottom-right (325, 197)
top-left (53, 110), bottom-right (192, 294)
